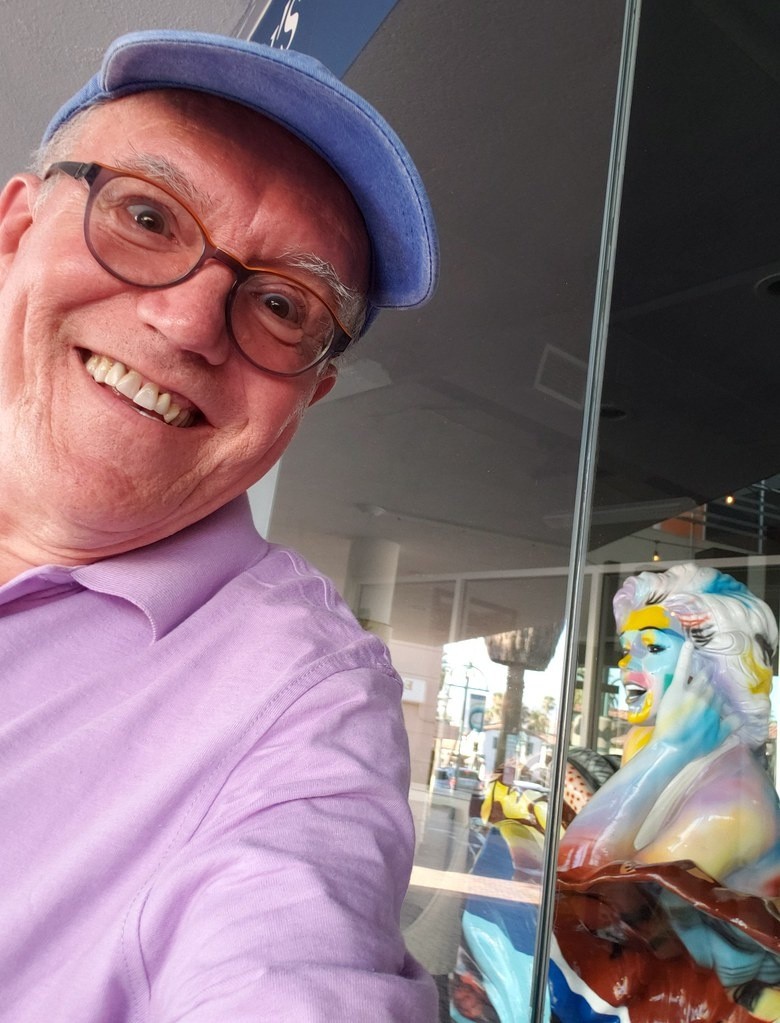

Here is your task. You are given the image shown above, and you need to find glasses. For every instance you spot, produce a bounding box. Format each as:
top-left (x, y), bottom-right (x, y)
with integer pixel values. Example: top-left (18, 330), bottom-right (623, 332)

top-left (42, 163), bottom-right (353, 379)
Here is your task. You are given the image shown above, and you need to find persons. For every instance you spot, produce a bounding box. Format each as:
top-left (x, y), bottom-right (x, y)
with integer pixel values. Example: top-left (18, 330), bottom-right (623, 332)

top-left (0, 31), bottom-right (440, 1023)
top-left (556, 563), bottom-right (780, 984)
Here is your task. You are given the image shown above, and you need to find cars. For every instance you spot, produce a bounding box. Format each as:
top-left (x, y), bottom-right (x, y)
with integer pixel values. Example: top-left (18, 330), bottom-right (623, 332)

top-left (431, 730), bottom-right (543, 799)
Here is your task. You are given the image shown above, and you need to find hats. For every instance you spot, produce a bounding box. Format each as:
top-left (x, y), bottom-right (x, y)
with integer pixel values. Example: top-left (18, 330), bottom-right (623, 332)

top-left (43, 29), bottom-right (439, 356)
